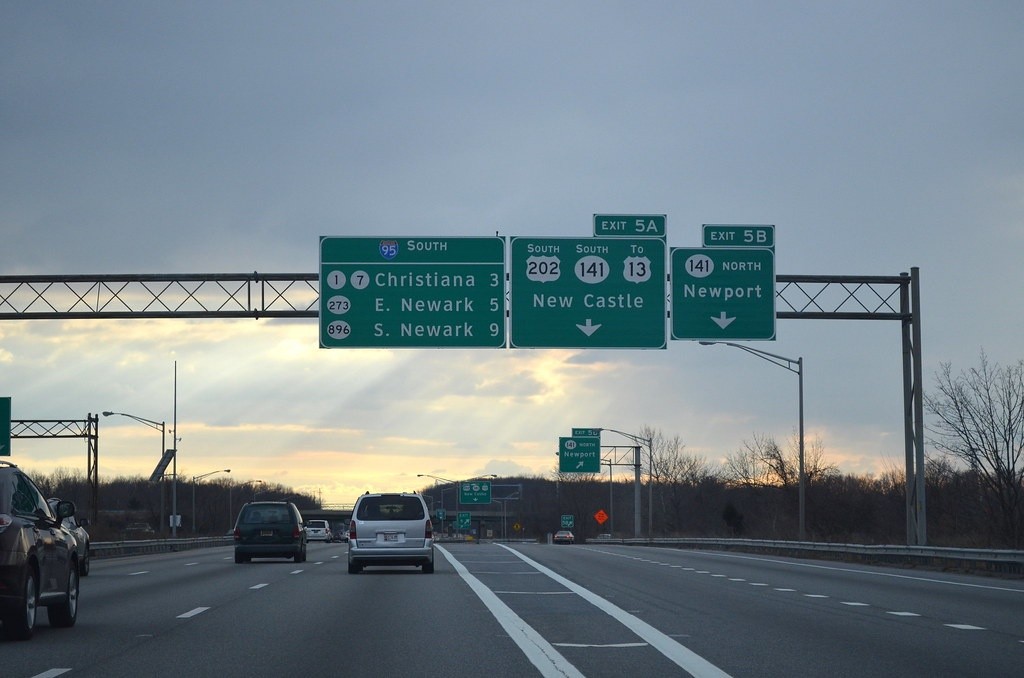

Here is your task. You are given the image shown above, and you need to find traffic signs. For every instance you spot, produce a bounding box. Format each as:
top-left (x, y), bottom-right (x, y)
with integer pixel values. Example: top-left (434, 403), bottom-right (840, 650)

top-left (509, 235), bottom-right (667, 350)
top-left (459, 481), bottom-right (492, 505)
top-left (669, 246), bottom-right (776, 341)
top-left (558, 437), bottom-right (601, 473)
top-left (457, 512), bottom-right (471, 528)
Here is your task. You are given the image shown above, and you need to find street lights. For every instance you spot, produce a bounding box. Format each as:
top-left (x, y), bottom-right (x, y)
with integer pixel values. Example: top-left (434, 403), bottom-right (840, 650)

top-left (593, 429), bottom-right (655, 547)
top-left (253, 488), bottom-right (281, 501)
top-left (417, 474), bottom-right (498, 539)
top-left (504, 492), bottom-right (519, 539)
top-left (229, 480), bottom-right (262, 535)
top-left (102, 411), bottom-right (165, 539)
top-left (192, 469), bottom-right (231, 533)
top-left (698, 340), bottom-right (806, 560)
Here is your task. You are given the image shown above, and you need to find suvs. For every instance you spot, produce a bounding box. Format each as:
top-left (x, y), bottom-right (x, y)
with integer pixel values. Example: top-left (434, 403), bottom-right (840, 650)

top-left (347, 491), bottom-right (435, 574)
top-left (47, 498), bottom-right (91, 577)
top-left (0, 461), bottom-right (81, 641)
top-left (234, 500), bottom-right (312, 564)
top-left (305, 520), bottom-right (331, 543)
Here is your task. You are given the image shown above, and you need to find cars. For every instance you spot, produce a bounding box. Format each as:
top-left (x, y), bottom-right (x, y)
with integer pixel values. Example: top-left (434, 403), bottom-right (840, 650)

top-left (330, 524), bottom-right (350, 543)
top-left (554, 530), bottom-right (575, 544)
top-left (597, 533), bottom-right (614, 539)
